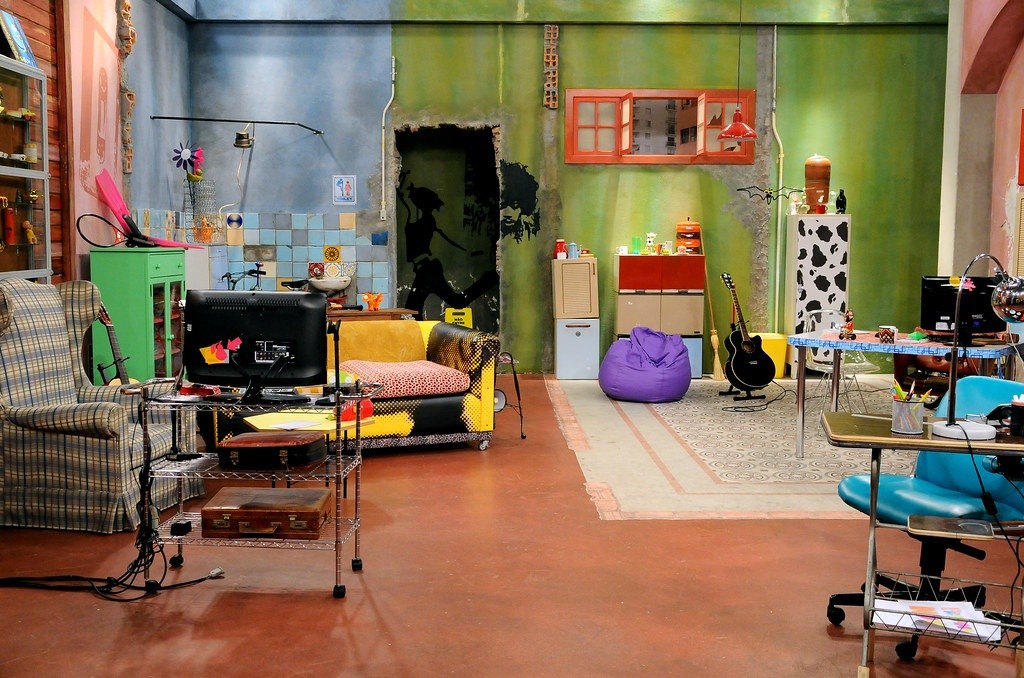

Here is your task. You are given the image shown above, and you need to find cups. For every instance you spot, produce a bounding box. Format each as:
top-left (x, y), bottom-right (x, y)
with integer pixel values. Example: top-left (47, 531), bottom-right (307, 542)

top-left (11, 153), bottom-right (26, 161)
top-left (632, 236), bottom-right (640, 254)
top-left (816, 204), bottom-right (828, 214)
top-left (556, 252), bottom-right (566, 259)
top-left (878, 325), bottom-right (899, 344)
top-left (999, 401), bottom-right (1024, 438)
top-left (616, 246), bottom-right (628, 255)
top-left (890, 399), bottom-right (924, 434)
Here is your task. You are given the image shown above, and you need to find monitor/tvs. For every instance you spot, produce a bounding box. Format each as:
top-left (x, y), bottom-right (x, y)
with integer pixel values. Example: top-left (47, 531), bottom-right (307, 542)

top-left (920, 275), bottom-right (1007, 348)
top-left (184, 289), bottom-right (327, 406)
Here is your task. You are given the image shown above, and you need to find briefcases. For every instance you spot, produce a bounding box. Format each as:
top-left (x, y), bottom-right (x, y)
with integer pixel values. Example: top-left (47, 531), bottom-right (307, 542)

top-left (217, 432), bottom-right (326, 471)
top-left (200, 486), bottom-right (331, 540)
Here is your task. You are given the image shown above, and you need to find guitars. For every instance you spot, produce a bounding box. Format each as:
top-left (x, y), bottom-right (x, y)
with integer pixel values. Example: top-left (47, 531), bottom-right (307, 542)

top-left (719, 272), bottom-right (776, 391)
top-left (95, 301), bottom-right (140, 386)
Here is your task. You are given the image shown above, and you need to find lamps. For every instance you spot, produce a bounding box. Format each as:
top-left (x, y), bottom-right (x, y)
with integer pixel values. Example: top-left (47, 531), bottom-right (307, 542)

top-left (717, 0), bottom-right (758, 142)
top-left (933, 253), bottom-right (1024, 440)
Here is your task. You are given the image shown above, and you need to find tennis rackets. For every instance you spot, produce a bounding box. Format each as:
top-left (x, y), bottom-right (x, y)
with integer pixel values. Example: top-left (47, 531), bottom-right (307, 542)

top-left (76, 213), bottom-right (154, 248)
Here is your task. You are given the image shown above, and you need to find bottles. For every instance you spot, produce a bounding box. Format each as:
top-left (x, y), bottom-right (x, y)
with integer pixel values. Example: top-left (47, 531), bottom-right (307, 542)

top-left (4, 204), bottom-right (14, 244)
top-left (325, 399), bottom-right (375, 421)
top-left (554, 239), bottom-right (568, 258)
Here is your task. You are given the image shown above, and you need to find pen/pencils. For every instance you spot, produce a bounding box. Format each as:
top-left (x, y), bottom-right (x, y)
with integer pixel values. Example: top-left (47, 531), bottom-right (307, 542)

top-left (890, 378), bottom-right (932, 432)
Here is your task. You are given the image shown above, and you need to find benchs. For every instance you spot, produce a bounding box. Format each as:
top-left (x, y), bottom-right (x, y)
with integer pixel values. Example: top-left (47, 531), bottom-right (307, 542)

top-left (196, 320), bottom-right (503, 469)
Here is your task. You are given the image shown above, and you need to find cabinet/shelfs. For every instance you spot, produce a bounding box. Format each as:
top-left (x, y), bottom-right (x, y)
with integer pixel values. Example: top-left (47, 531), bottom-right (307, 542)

top-left (89, 247), bottom-right (187, 386)
top-left (121, 378), bottom-right (384, 599)
top-left (0, 54), bottom-right (54, 285)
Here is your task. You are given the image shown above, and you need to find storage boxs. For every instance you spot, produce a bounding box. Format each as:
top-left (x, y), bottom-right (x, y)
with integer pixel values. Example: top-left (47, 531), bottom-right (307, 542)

top-left (550, 213), bottom-right (852, 379)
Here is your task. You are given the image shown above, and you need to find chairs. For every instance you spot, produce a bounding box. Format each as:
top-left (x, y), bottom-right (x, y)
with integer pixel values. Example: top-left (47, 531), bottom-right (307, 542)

top-left (892, 326), bottom-right (997, 401)
top-left (827, 375), bottom-right (1024, 661)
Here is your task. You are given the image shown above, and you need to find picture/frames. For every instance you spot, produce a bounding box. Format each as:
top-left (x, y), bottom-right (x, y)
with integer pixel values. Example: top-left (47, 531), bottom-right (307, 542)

top-left (0, 8), bottom-right (41, 69)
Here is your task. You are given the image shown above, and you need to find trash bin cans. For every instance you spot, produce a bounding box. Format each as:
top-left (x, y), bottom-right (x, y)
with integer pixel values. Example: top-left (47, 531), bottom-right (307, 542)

top-left (749, 332), bottom-right (788, 379)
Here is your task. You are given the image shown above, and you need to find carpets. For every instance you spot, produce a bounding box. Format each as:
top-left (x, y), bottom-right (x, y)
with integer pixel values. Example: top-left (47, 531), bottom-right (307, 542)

top-left (543, 374), bottom-right (941, 521)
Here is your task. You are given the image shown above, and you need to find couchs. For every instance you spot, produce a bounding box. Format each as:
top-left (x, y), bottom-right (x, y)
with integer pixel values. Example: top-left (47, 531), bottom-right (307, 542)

top-left (0, 277), bottom-right (207, 534)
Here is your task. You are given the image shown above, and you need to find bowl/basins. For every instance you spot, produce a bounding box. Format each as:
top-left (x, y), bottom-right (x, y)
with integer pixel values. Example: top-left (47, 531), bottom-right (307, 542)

top-left (309, 276), bottom-right (351, 291)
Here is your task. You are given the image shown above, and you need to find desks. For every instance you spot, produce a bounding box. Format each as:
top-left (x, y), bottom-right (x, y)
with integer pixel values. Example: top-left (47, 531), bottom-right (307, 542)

top-left (821, 411), bottom-right (1024, 678)
top-left (244, 408), bottom-right (375, 498)
top-left (326, 307), bottom-right (419, 322)
top-left (786, 328), bottom-right (1024, 458)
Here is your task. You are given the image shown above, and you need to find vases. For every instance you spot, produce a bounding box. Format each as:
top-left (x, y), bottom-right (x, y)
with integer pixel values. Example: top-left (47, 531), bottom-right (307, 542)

top-left (183, 178), bottom-right (219, 245)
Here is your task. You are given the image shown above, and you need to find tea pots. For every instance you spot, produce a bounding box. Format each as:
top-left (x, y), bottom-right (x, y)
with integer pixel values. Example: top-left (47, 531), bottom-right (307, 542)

top-left (563, 241), bottom-right (582, 258)
